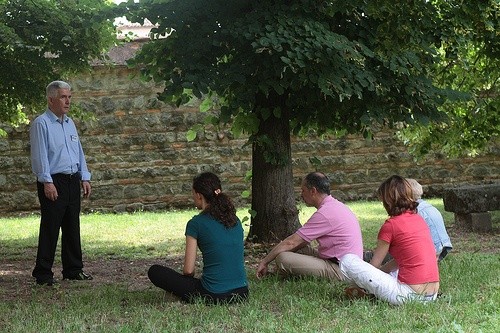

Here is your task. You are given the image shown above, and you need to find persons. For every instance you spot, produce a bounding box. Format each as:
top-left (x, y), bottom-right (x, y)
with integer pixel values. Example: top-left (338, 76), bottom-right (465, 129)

top-left (255, 172), bottom-right (363, 281)
top-left (30, 80), bottom-right (91, 285)
top-left (148, 172), bottom-right (248, 307)
top-left (364, 177), bottom-right (453, 263)
top-left (340, 175), bottom-right (439, 306)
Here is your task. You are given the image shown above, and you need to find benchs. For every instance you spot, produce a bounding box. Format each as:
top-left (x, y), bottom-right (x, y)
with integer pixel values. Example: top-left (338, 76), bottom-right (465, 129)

top-left (443, 184), bottom-right (500, 232)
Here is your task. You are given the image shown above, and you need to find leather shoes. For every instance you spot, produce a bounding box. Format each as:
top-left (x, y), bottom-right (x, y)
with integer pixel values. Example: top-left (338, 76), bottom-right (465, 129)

top-left (35, 279), bottom-right (61, 287)
top-left (63, 272), bottom-right (93, 280)
